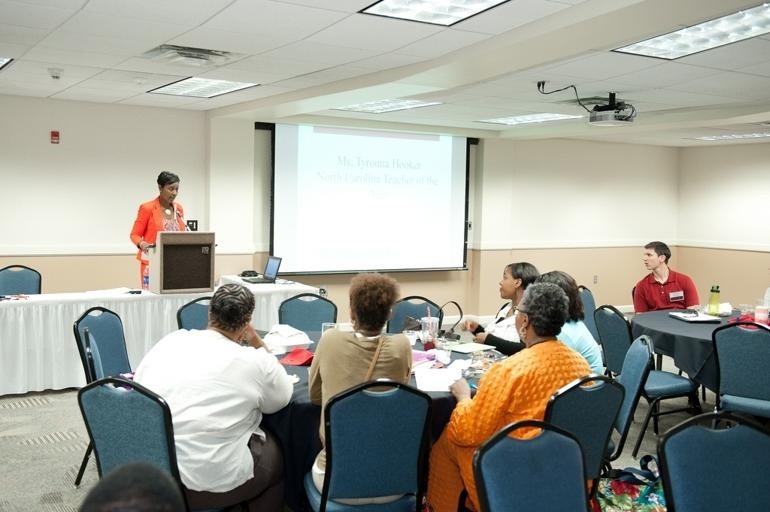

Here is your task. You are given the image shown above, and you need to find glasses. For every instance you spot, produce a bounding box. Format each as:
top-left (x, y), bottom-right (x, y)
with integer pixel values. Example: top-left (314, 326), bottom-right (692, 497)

top-left (512, 306), bottom-right (534, 316)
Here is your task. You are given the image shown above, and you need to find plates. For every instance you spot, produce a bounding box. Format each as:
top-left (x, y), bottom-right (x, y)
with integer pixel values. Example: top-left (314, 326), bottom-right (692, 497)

top-left (669, 310), bottom-right (721, 323)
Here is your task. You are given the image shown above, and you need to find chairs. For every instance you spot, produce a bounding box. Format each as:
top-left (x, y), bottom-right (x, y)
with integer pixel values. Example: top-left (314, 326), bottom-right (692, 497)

top-left (543, 373), bottom-right (625, 499)
top-left (632, 286), bottom-right (635, 300)
top-left (472, 419), bottom-right (592, 512)
top-left (72, 305), bottom-right (134, 488)
top-left (175, 295), bottom-right (213, 331)
top-left (601, 333), bottom-right (655, 476)
top-left (3, 265), bottom-right (43, 298)
top-left (277, 290), bottom-right (338, 333)
top-left (75, 374), bottom-right (192, 511)
top-left (303, 377), bottom-right (436, 512)
top-left (654, 409), bottom-right (770, 512)
top-left (576, 285), bottom-right (608, 368)
top-left (385, 296), bottom-right (443, 336)
top-left (710, 321), bottom-right (770, 429)
top-left (592, 304), bottom-right (700, 459)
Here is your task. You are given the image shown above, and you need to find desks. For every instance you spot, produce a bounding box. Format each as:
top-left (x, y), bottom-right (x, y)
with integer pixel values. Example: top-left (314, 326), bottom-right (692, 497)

top-left (0, 274), bottom-right (328, 400)
top-left (241, 330), bottom-right (506, 512)
top-left (631, 308), bottom-right (770, 415)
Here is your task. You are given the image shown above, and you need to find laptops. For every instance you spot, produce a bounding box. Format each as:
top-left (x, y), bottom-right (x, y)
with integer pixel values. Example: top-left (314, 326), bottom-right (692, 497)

top-left (243, 255), bottom-right (281, 284)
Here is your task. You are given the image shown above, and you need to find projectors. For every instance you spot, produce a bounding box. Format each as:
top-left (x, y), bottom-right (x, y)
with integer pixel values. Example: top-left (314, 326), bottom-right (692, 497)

top-left (589, 102), bottom-right (637, 128)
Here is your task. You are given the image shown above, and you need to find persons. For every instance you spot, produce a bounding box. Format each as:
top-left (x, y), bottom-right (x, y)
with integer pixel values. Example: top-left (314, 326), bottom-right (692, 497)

top-left (309, 272), bottom-right (414, 504)
top-left (134, 285), bottom-right (293, 512)
top-left (425, 283), bottom-right (594, 512)
top-left (461, 263), bottom-right (539, 343)
top-left (534, 270), bottom-right (607, 387)
top-left (78, 463), bottom-right (187, 512)
top-left (635, 243), bottom-right (704, 415)
top-left (130, 170), bottom-right (194, 289)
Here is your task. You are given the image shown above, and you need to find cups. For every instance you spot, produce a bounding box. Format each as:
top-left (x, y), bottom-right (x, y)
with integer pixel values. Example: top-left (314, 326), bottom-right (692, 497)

top-left (472, 351), bottom-right (484, 365)
top-left (739, 304), bottom-right (748, 315)
top-left (421, 317), bottom-right (440, 351)
top-left (402, 330), bottom-right (419, 346)
top-left (755, 306), bottom-right (768, 324)
top-left (322, 323), bottom-right (336, 335)
top-left (708, 285), bottom-right (721, 316)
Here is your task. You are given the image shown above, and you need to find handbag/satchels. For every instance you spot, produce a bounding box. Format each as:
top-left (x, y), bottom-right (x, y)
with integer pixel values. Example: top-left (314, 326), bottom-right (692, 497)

top-left (596, 453), bottom-right (667, 512)
